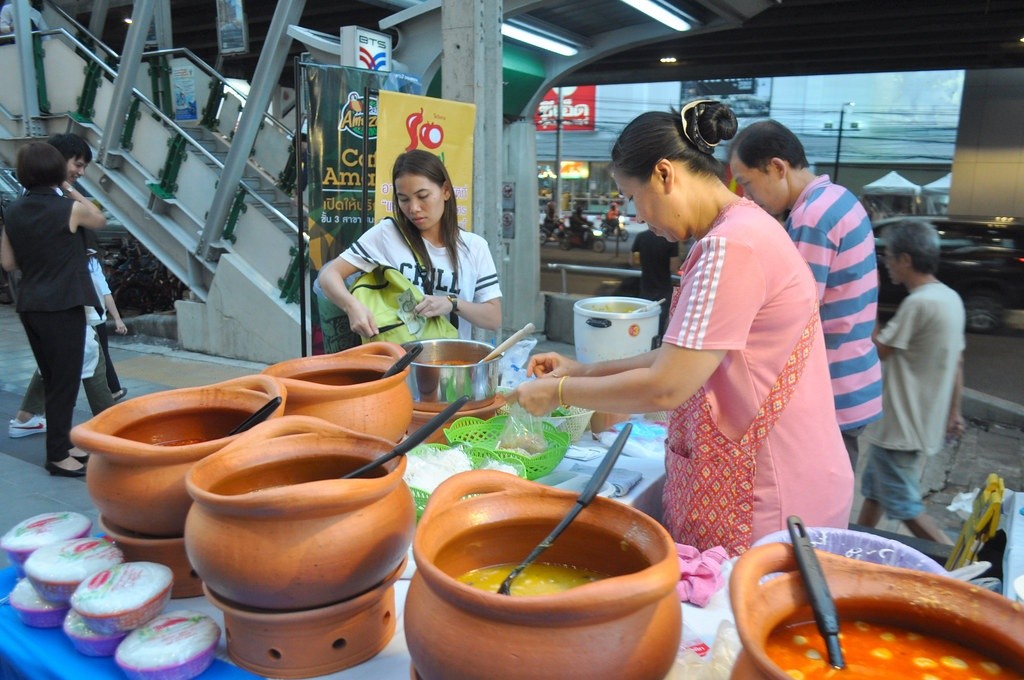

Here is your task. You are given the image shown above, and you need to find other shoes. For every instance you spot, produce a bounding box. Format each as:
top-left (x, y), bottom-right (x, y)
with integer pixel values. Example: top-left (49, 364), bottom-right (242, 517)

top-left (111, 388), bottom-right (128, 401)
top-left (45, 461), bottom-right (87, 475)
top-left (66, 453), bottom-right (90, 461)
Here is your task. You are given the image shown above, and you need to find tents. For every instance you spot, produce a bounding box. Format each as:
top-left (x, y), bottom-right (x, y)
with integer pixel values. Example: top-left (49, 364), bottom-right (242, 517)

top-left (863, 170), bottom-right (921, 200)
top-left (923, 171), bottom-right (951, 195)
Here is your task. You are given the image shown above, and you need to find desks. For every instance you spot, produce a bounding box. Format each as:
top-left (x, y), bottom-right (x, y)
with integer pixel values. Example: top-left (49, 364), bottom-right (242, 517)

top-left (0, 357), bottom-right (738, 680)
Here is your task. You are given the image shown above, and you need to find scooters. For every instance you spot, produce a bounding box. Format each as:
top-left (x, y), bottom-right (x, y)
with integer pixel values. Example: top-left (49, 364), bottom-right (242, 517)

top-left (540, 211), bottom-right (629, 253)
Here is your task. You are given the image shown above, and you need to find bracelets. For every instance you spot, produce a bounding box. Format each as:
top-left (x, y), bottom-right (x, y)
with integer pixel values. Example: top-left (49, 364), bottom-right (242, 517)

top-left (559, 375), bottom-right (570, 409)
top-left (64, 186), bottom-right (74, 196)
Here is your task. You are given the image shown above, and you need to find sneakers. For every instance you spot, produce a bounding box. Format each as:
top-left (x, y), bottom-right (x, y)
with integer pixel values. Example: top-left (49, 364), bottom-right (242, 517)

top-left (9, 417), bottom-right (47, 438)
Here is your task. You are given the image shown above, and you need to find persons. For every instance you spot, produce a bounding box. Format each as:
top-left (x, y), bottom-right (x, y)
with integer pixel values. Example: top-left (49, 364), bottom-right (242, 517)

top-left (631, 223), bottom-right (678, 346)
top-left (856, 222), bottom-right (966, 549)
top-left (544, 201), bottom-right (565, 239)
top-left (607, 202), bottom-right (620, 236)
top-left (80, 227), bottom-right (127, 402)
top-left (320, 149), bottom-right (502, 352)
top-left (569, 207), bottom-right (593, 243)
top-left (9, 132), bottom-right (116, 438)
top-left (294, 134), bottom-right (309, 191)
top-left (597, 191), bottom-right (625, 205)
top-left (517, 98), bottom-right (854, 561)
top-left (0, 140), bottom-right (106, 477)
top-left (727, 119), bottom-right (883, 475)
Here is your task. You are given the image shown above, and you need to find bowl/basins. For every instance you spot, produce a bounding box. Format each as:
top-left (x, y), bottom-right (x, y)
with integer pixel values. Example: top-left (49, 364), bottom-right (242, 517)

top-left (1, 511), bottom-right (221, 680)
top-left (400, 339), bottom-right (505, 412)
top-left (407, 392), bottom-right (505, 448)
top-left (751, 526), bottom-right (949, 583)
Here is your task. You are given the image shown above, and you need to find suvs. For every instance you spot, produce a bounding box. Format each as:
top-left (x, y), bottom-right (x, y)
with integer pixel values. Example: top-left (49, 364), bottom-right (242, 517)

top-left (870, 213), bottom-right (1024, 337)
top-left (0, 166), bottom-right (134, 303)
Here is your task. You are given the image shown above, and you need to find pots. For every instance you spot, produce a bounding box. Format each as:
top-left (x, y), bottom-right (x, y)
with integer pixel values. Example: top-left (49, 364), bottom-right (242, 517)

top-left (260, 340), bottom-right (413, 442)
top-left (184, 414), bottom-right (416, 608)
top-left (573, 296), bottom-right (662, 363)
top-left (69, 373), bottom-right (287, 536)
top-left (404, 467), bottom-right (682, 679)
top-left (729, 541), bottom-right (1024, 680)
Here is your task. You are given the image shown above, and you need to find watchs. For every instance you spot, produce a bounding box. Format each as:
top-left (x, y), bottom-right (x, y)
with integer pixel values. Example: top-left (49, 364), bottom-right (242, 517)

top-left (448, 294), bottom-right (457, 312)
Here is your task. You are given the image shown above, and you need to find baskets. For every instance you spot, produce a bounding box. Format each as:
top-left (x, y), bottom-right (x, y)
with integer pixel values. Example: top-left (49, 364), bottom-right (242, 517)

top-left (404, 384), bottom-right (591, 525)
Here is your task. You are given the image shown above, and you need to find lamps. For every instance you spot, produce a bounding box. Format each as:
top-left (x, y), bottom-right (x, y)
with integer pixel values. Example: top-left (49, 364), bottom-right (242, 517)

top-left (623, 0), bottom-right (701, 31)
top-left (500, 12), bottom-right (593, 56)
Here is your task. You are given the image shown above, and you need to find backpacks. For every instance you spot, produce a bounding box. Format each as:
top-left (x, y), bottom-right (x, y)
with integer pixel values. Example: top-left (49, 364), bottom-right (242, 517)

top-left (346, 267), bottom-right (458, 345)
